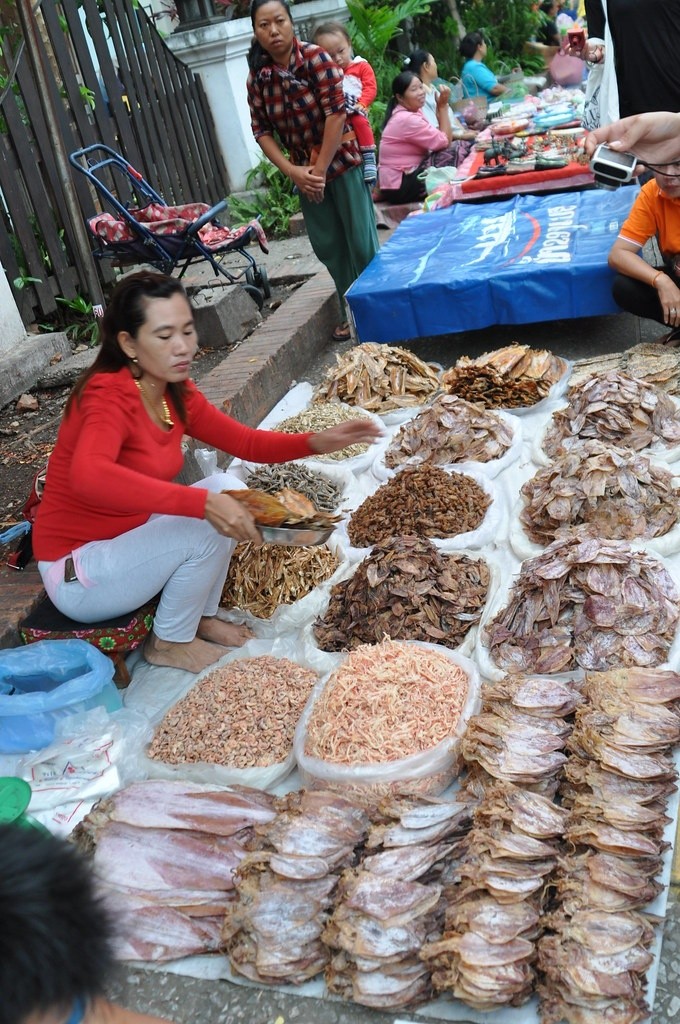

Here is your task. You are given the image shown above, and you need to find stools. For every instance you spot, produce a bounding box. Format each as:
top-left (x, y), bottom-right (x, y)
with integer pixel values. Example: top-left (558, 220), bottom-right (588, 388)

top-left (19, 589), bottom-right (163, 690)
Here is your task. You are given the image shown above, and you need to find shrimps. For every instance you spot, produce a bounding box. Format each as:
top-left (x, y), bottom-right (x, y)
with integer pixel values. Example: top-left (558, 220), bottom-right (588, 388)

top-left (150, 631), bottom-right (320, 770)
top-left (309, 631), bottom-right (472, 811)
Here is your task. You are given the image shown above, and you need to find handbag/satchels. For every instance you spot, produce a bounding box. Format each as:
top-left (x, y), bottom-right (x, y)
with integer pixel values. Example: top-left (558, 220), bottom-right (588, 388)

top-left (448, 76), bottom-right (486, 125)
top-left (491, 58), bottom-right (525, 89)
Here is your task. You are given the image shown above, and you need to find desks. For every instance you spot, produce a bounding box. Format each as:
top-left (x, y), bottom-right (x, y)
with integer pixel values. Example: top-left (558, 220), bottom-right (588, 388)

top-left (447, 88), bottom-right (595, 205)
top-left (342, 183), bottom-right (641, 345)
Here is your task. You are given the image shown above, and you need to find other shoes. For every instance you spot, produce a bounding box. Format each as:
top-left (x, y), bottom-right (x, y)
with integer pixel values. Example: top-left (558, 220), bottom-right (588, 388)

top-left (657, 329), bottom-right (680, 347)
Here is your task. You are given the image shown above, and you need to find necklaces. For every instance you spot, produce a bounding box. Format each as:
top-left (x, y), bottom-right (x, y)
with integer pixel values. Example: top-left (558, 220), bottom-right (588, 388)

top-left (133, 378), bottom-right (174, 426)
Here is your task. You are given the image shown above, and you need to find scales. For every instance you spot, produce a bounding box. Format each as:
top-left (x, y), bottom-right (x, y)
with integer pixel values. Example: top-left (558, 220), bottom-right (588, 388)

top-left (0, 776), bottom-right (54, 837)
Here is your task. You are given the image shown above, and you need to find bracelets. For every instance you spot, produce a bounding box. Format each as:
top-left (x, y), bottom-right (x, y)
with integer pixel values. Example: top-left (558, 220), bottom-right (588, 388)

top-left (652, 270), bottom-right (664, 287)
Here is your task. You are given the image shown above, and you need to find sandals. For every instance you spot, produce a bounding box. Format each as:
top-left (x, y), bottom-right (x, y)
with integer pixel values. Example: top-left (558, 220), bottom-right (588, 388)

top-left (332, 321), bottom-right (351, 341)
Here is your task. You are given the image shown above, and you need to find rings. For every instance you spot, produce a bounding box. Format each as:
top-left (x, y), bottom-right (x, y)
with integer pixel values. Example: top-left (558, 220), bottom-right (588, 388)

top-left (670, 308), bottom-right (677, 315)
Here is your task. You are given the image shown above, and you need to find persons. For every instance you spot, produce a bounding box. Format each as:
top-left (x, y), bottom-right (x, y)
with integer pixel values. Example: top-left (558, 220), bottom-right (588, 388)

top-left (310, 18), bottom-right (379, 187)
top-left (603, 162), bottom-right (680, 347)
top-left (399, 50), bottom-right (477, 153)
top-left (377, 71), bottom-right (471, 205)
top-left (519, 0), bottom-right (680, 133)
top-left (240, 0), bottom-right (381, 342)
top-left (580, 110), bottom-right (680, 177)
top-left (31, 267), bottom-right (385, 675)
top-left (456, 28), bottom-right (528, 104)
top-left (0, 816), bottom-right (180, 1023)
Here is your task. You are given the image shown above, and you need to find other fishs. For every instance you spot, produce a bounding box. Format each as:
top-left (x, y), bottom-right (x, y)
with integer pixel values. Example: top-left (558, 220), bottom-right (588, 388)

top-left (65, 666), bottom-right (680, 1024)
top-left (214, 341), bottom-right (680, 680)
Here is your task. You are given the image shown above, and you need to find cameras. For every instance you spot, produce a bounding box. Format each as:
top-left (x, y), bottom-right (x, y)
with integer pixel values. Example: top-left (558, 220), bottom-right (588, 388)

top-left (589, 141), bottom-right (638, 192)
top-left (568, 29), bottom-right (586, 51)
